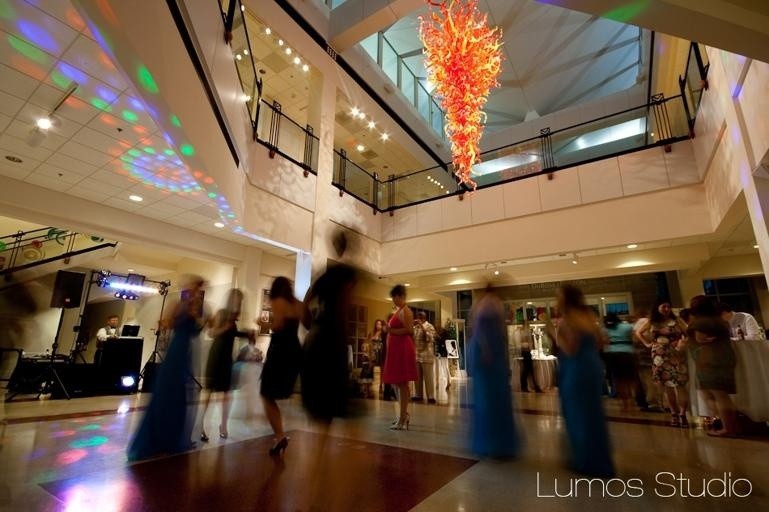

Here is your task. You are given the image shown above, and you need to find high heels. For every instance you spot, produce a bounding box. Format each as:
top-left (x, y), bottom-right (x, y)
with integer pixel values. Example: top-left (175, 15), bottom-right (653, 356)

top-left (269, 437), bottom-right (287, 455)
top-left (390, 413), bottom-right (410, 431)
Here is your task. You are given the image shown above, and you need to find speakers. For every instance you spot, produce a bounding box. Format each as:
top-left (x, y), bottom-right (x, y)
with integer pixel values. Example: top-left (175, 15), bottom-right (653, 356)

top-left (51, 269), bottom-right (85, 309)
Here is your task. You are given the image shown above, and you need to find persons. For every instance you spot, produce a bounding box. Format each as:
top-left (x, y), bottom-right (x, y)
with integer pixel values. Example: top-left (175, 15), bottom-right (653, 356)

top-left (687, 293), bottom-right (739, 438)
top-left (195, 287), bottom-right (256, 442)
top-left (125, 290), bottom-right (207, 455)
top-left (636, 297), bottom-right (691, 426)
top-left (536, 283), bottom-right (616, 480)
top-left (380, 282), bottom-right (415, 430)
top-left (94, 314), bottom-right (119, 362)
top-left (463, 272), bottom-right (521, 464)
top-left (368, 309), bottom-right (688, 428)
top-left (298, 225), bottom-right (372, 487)
top-left (717, 302), bottom-right (762, 343)
top-left (252, 277), bottom-right (306, 456)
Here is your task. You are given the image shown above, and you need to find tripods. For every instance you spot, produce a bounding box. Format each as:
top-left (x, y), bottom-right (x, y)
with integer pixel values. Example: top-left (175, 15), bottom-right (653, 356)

top-left (6, 310), bottom-right (73, 402)
top-left (139, 294), bottom-right (170, 380)
top-left (66, 282), bottom-right (99, 364)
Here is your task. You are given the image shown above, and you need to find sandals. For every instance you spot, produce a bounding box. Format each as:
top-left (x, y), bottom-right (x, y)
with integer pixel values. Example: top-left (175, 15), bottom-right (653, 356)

top-left (639, 405), bottom-right (739, 438)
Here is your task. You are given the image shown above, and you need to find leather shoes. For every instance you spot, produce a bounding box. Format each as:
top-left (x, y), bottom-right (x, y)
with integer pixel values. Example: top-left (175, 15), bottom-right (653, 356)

top-left (200, 431), bottom-right (208, 441)
top-left (220, 426), bottom-right (227, 438)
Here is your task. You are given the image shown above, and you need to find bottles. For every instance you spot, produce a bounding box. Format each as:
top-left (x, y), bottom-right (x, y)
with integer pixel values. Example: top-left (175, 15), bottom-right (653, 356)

top-left (736, 324), bottom-right (743, 339)
top-left (755, 324), bottom-right (763, 340)
top-left (46, 348), bottom-right (50, 358)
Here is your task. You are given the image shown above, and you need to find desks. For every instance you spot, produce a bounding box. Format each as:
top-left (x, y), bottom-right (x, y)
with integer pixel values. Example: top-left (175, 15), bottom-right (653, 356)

top-left (512, 355), bottom-right (558, 392)
top-left (687, 340), bottom-right (769, 422)
